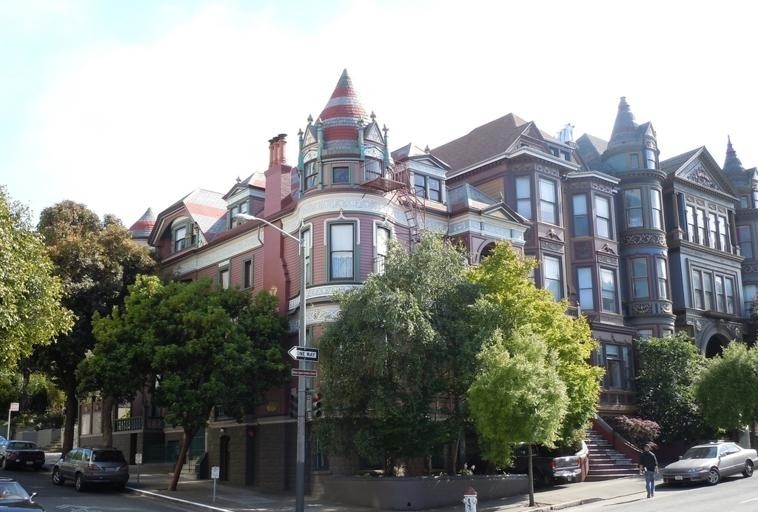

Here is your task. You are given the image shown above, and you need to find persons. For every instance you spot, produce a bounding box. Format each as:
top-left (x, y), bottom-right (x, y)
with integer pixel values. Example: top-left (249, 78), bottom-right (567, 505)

top-left (638, 443), bottom-right (659, 498)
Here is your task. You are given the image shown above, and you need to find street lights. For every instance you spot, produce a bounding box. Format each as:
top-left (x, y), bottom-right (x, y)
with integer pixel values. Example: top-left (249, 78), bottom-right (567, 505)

top-left (232, 213), bottom-right (307, 511)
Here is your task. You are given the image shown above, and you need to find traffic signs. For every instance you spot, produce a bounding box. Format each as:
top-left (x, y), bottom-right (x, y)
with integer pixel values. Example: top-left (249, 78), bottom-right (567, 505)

top-left (288, 345), bottom-right (320, 362)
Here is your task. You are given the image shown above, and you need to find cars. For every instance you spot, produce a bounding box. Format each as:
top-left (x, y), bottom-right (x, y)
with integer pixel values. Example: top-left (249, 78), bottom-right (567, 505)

top-left (0, 436), bottom-right (45, 471)
top-left (663, 440), bottom-right (757, 487)
top-left (0, 476), bottom-right (45, 512)
top-left (51, 446), bottom-right (129, 492)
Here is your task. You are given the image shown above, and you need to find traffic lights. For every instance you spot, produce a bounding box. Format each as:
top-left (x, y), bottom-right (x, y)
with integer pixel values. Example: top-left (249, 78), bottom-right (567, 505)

top-left (312, 392), bottom-right (322, 419)
top-left (287, 393), bottom-right (298, 419)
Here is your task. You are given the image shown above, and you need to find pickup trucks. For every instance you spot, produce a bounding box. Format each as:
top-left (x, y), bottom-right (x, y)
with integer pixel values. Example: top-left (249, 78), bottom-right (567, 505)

top-left (502, 441), bottom-right (582, 487)
